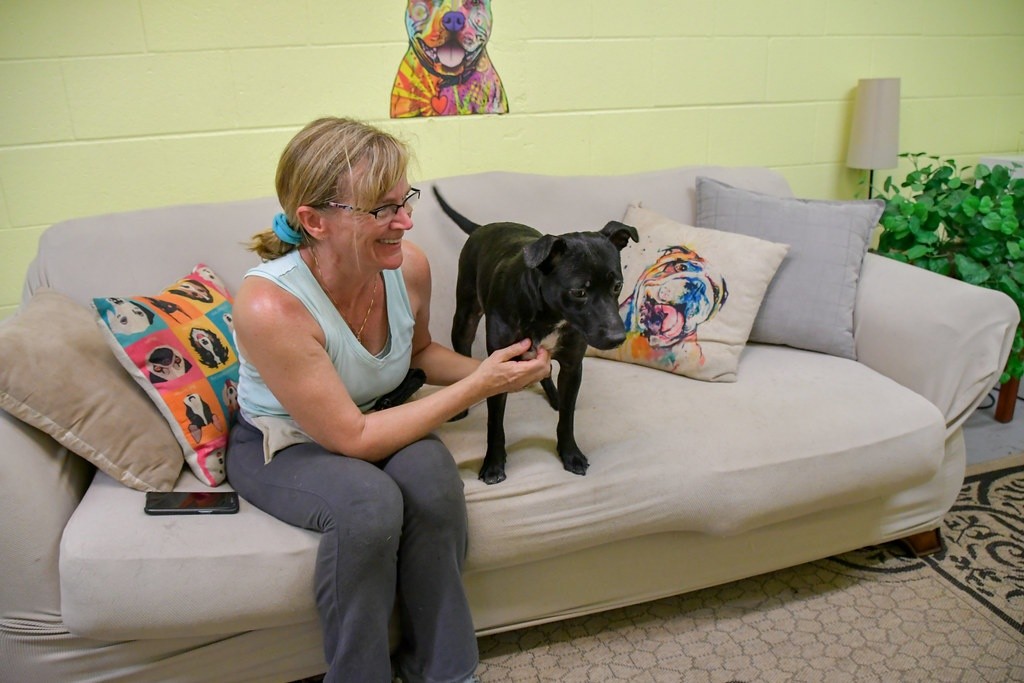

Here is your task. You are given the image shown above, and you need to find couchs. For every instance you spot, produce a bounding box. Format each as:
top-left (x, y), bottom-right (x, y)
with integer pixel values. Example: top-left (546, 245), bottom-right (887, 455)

top-left (0, 166), bottom-right (1021, 683)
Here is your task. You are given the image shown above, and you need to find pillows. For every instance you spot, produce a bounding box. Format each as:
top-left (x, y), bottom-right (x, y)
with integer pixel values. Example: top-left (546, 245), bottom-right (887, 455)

top-left (0, 287), bottom-right (183, 495)
top-left (86, 260), bottom-right (242, 486)
top-left (688, 178), bottom-right (888, 360)
top-left (582, 203), bottom-right (790, 386)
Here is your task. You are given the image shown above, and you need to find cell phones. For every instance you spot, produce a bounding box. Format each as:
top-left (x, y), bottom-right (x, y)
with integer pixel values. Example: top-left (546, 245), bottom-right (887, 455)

top-left (144, 491), bottom-right (240, 514)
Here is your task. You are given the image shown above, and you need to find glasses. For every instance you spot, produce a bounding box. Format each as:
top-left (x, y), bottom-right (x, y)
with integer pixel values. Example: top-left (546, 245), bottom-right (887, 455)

top-left (313, 188), bottom-right (421, 226)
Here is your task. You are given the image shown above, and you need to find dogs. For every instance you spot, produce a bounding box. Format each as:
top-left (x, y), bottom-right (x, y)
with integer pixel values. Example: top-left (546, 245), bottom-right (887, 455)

top-left (431, 185), bottom-right (640, 486)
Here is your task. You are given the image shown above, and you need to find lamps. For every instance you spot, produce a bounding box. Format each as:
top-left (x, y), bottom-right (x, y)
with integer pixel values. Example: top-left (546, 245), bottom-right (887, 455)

top-left (847, 77), bottom-right (902, 198)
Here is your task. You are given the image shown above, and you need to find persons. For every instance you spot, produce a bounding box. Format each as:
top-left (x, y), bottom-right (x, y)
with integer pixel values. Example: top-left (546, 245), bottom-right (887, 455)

top-left (223, 117), bottom-right (552, 683)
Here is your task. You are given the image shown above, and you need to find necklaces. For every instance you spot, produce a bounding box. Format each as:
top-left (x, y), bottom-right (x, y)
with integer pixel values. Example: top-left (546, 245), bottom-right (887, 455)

top-left (311, 245), bottom-right (378, 344)
